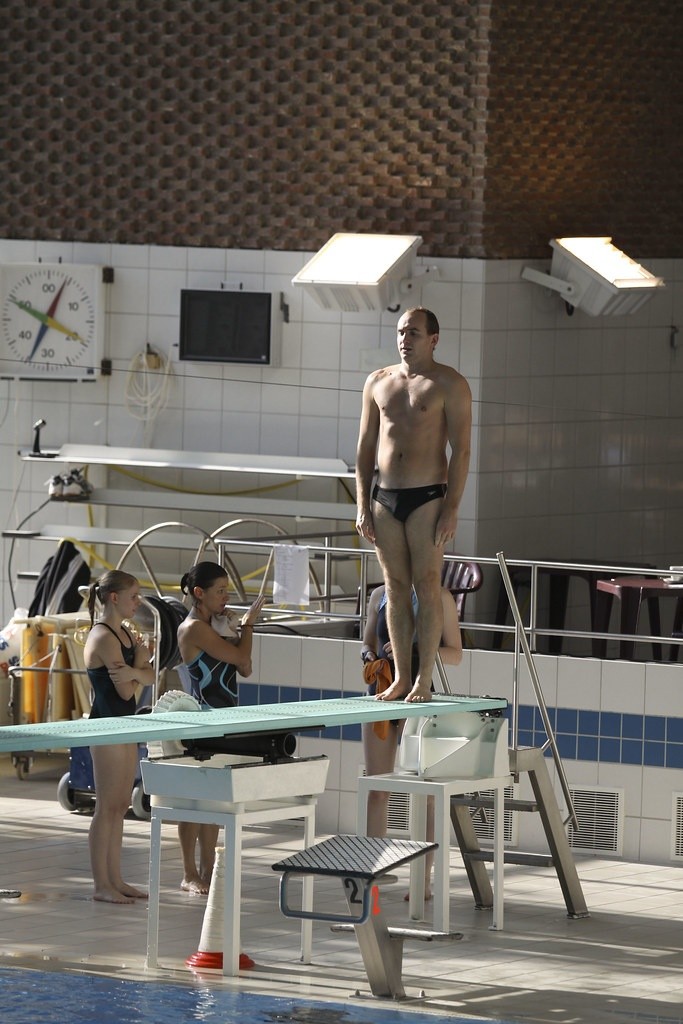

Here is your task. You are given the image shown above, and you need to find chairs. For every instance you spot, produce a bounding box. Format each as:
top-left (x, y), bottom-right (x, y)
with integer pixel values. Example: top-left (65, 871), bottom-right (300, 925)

top-left (353, 550), bottom-right (483, 648)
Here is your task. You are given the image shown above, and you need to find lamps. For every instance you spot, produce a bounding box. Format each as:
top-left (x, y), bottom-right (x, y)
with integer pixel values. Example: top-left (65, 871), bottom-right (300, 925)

top-left (519, 236), bottom-right (667, 319)
top-left (290, 232), bottom-right (424, 313)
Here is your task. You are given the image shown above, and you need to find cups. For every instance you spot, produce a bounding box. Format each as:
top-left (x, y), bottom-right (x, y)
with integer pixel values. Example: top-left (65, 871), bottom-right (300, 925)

top-left (670, 566), bottom-right (683, 580)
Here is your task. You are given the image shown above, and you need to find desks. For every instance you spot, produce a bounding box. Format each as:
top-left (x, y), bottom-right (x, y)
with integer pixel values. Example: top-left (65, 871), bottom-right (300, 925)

top-left (491, 556), bottom-right (663, 662)
top-left (592, 578), bottom-right (683, 664)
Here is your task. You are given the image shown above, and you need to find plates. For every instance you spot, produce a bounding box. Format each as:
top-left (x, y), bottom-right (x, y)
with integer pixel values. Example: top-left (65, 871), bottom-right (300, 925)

top-left (663, 578), bottom-right (683, 583)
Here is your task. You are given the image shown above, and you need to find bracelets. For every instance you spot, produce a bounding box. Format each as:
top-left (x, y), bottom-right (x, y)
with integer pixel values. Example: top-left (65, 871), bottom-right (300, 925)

top-left (241, 625), bottom-right (253, 629)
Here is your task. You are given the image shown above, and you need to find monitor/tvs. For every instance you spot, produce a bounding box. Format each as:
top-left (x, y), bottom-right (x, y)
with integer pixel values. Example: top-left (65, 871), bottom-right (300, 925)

top-left (178, 288), bottom-right (271, 364)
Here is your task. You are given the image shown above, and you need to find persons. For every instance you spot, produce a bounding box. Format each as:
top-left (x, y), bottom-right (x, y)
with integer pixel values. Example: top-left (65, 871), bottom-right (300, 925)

top-left (83, 570), bottom-right (156, 904)
top-left (176, 561), bottom-right (265, 894)
top-left (360, 584), bottom-right (462, 901)
top-left (355, 308), bottom-right (473, 703)
